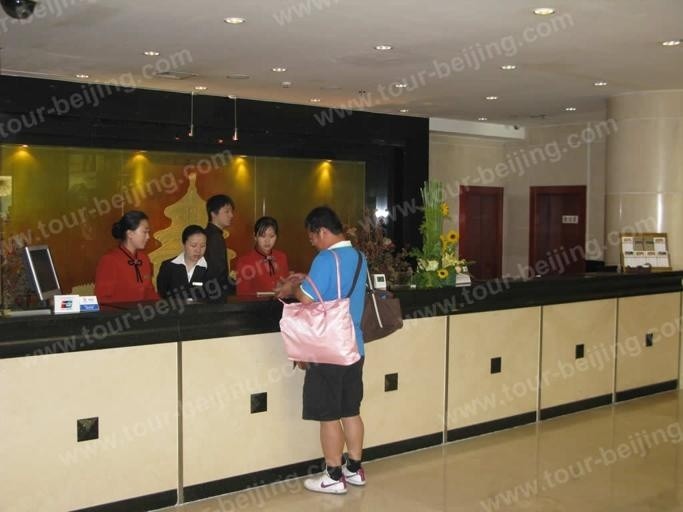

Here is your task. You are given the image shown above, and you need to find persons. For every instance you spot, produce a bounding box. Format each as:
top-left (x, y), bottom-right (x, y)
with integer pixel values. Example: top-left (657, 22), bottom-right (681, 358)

top-left (92, 210), bottom-right (161, 304)
top-left (236, 216), bottom-right (291, 300)
top-left (203, 194), bottom-right (237, 292)
top-left (273, 206), bottom-right (368, 494)
top-left (157, 225), bottom-right (228, 299)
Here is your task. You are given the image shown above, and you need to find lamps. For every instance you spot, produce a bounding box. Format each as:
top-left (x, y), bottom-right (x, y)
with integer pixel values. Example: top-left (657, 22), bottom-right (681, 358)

top-left (232, 95), bottom-right (238, 141)
top-left (189, 89), bottom-right (194, 138)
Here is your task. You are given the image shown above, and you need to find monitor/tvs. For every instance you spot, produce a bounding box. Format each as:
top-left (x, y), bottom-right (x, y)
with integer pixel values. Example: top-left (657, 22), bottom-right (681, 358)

top-left (22, 244), bottom-right (62, 310)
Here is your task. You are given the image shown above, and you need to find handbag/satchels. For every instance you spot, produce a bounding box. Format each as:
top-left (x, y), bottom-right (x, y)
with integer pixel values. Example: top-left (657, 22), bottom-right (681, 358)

top-left (278, 248), bottom-right (361, 373)
top-left (361, 248), bottom-right (403, 345)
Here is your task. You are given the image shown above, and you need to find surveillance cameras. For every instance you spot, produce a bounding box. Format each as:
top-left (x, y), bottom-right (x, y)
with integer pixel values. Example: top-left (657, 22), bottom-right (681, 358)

top-left (0, 0), bottom-right (39, 20)
top-left (514, 125), bottom-right (519, 129)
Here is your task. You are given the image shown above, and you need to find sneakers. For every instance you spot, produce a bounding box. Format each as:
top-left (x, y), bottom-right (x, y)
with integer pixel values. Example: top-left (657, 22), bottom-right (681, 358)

top-left (303, 473), bottom-right (347, 495)
top-left (316, 461), bottom-right (368, 486)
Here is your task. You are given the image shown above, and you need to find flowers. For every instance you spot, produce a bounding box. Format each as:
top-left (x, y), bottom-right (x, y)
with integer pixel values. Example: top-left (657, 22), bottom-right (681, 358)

top-left (2, 236), bottom-right (37, 314)
top-left (344, 207), bottom-right (409, 284)
top-left (410, 181), bottom-right (463, 290)
